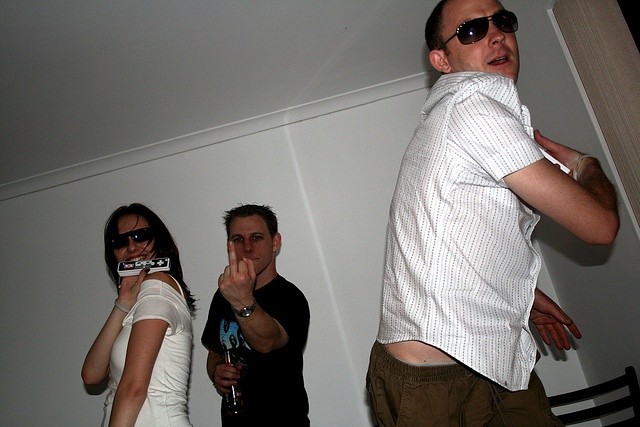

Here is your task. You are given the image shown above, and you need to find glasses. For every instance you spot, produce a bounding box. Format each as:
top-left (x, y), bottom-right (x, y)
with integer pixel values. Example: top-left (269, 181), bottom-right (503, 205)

top-left (438, 11), bottom-right (518, 50)
top-left (113, 227), bottom-right (157, 250)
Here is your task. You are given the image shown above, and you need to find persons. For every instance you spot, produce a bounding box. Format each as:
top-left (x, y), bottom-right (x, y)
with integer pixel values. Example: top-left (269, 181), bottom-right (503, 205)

top-left (365, 0), bottom-right (620, 427)
top-left (81, 203), bottom-right (200, 427)
top-left (200, 201), bottom-right (311, 427)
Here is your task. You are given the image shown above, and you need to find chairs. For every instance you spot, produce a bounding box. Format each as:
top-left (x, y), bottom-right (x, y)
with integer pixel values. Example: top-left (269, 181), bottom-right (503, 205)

top-left (548, 365), bottom-right (639, 426)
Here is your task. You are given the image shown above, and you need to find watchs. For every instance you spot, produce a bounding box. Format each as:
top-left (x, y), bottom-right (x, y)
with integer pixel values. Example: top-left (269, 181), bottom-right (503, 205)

top-left (232, 297), bottom-right (257, 317)
